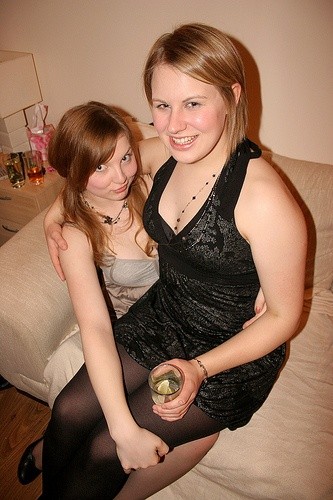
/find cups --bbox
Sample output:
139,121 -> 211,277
3,154 -> 25,189
22,150 -> 45,186
148,363 -> 185,406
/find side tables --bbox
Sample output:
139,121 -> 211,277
0,161 -> 64,247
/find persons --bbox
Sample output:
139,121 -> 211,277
42,101 -> 268,499
19,22 -> 306,500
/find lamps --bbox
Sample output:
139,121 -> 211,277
0,50 -> 43,153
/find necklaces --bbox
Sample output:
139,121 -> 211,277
173,156 -> 223,254
81,195 -> 128,225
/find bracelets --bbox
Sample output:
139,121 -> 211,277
194,359 -> 208,383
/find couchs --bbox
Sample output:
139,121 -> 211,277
0,117 -> 333,500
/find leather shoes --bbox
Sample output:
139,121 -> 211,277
17,435 -> 42,486
